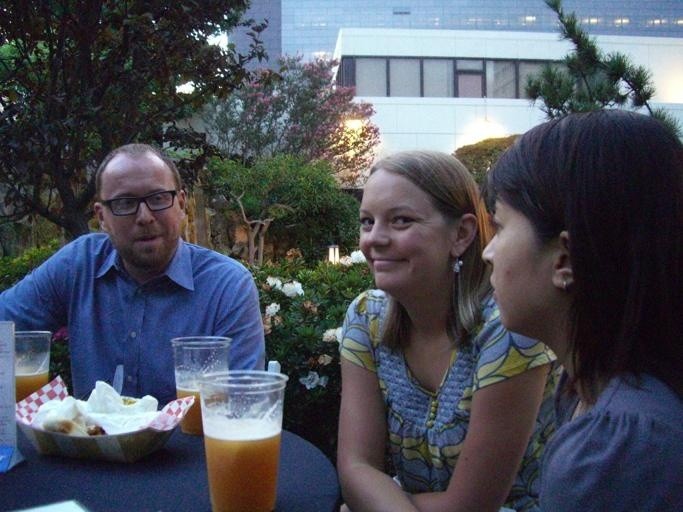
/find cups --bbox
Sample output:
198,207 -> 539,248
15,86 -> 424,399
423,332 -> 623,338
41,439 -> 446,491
11,331 -> 51,402
198,370 -> 286,511
171,334 -> 233,438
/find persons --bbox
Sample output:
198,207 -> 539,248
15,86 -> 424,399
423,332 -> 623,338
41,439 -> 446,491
480,109 -> 680,512
334,150 -> 567,512
0,142 -> 267,409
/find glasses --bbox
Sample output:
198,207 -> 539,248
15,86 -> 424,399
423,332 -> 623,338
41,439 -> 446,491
101,188 -> 180,217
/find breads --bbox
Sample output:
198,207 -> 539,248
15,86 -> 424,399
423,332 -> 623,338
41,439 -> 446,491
32,381 -> 159,439
